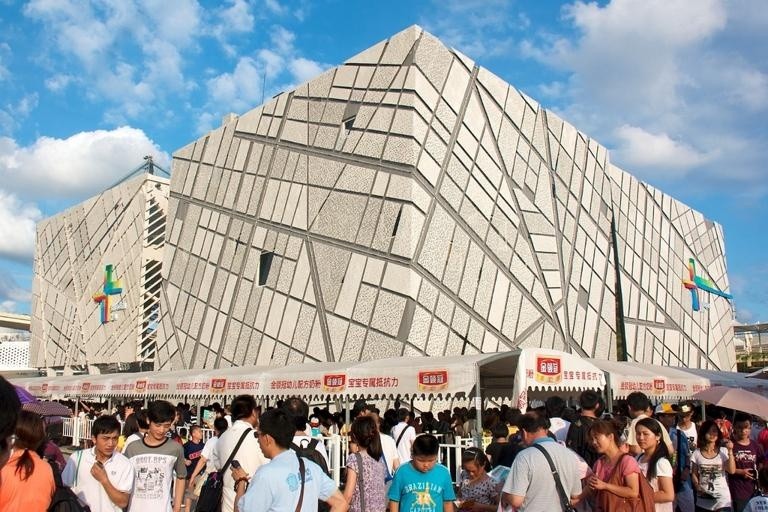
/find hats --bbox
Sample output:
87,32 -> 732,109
654,403 -> 679,414
679,401 -> 696,417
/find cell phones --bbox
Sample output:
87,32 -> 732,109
231,460 -> 240,468
97,461 -> 103,468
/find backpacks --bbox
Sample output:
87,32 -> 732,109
298,437 -> 329,479
47,461 -> 90,512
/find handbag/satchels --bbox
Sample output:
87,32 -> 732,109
674,470 -> 686,493
193,468 -> 224,512
594,451 -> 656,512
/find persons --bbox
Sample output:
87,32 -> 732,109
1,376 -> 768,511
